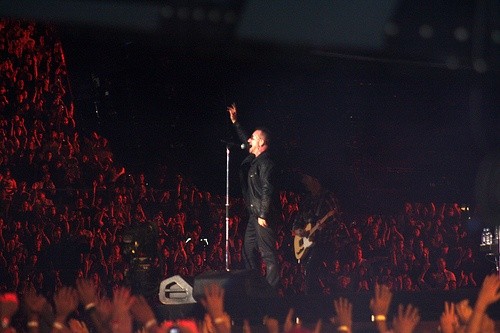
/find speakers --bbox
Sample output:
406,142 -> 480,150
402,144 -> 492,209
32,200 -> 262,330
158,269 -> 274,305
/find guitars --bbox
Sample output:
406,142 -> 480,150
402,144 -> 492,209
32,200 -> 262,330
294,208 -> 337,260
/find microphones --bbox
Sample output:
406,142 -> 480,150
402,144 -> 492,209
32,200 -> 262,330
220,139 -> 246,149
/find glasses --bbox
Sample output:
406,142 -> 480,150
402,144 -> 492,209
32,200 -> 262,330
251,135 -> 259,141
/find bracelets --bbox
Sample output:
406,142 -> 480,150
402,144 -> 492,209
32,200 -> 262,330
27,321 -> 39,326
336,326 -> 352,331
145,319 -> 154,328
213,317 -> 227,323
51,321 -> 62,330
85,303 -> 95,307
370,315 -> 386,321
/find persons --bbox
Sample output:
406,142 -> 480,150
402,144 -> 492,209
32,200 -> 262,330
226,102 -> 283,285
0,19 -> 500,333
